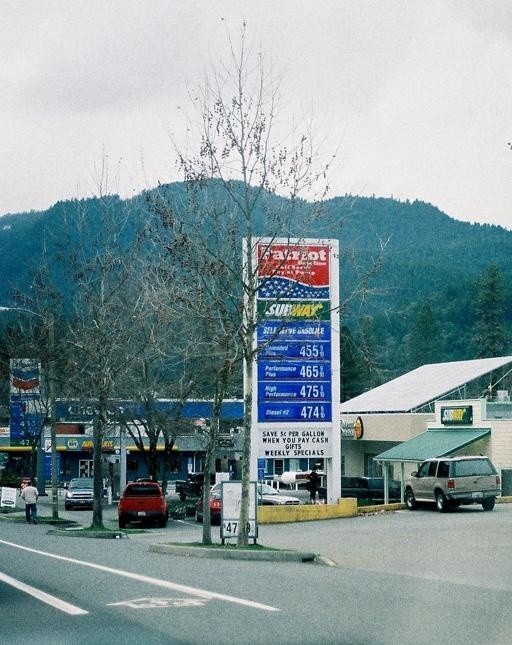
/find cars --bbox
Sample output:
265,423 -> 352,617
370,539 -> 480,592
194,482 -> 299,521
64,471 -> 95,510
118,478 -> 167,528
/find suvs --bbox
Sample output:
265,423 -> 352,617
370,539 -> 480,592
405,456 -> 500,512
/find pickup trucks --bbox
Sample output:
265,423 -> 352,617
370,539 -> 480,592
319,476 -> 401,505
176,472 -> 215,500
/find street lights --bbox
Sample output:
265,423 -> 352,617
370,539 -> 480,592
1,306 -> 57,519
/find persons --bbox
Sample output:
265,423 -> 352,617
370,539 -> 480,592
307,464 -> 319,500
21,482 -> 39,524
228,465 -> 235,480
